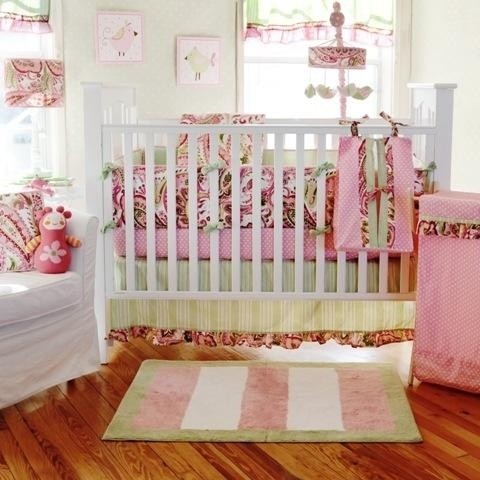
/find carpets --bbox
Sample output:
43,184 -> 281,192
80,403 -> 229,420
102,359 -> 422,443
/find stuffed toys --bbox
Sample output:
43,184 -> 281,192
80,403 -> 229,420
304,62 -> 374,104
24,205 -> 81,275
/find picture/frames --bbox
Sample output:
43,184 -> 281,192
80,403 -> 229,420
174,37 -> 224,85
94,11 -> 145,64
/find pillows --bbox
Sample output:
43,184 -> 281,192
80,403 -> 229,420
0,192 -> 44,268
177,112 -> 265,166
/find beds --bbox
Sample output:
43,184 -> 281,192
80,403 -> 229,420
100,116 -> 438,367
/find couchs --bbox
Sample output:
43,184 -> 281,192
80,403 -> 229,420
0,206 -> 101,409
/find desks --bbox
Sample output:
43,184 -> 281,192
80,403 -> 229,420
408,190 -> 480,394
